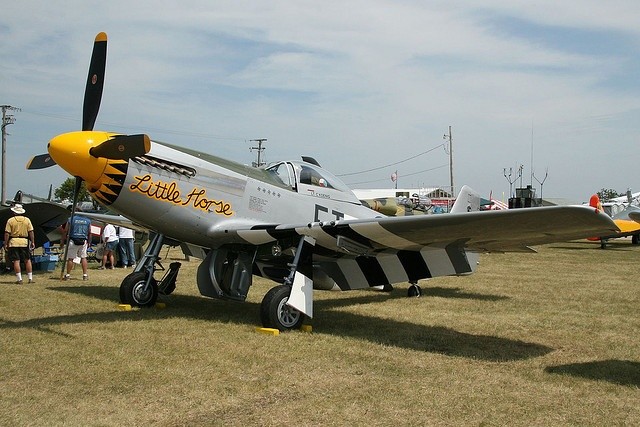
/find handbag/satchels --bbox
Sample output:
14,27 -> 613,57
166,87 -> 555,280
28,240 -> 34,253
70,237 -> 88,245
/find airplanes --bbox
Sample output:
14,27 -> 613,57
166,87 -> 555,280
595,187 -> 640,249
21,185 -> 101,212
26,31 -> 621,328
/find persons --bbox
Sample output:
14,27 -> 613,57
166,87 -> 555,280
119,215 -> 137,268
5,203 -> 36,285
59,207 -> 91,280
98,222 -> 118,270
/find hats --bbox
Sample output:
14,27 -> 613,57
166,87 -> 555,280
10,204 -> 26,214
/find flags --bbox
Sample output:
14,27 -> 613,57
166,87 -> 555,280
391,172 -> 397,182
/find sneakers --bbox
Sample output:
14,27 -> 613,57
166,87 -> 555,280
82,273 -> 89,281
28,279 -> 36,284
62,274 -> 70,281
15,280 -> 23,284
97,266 -> 106,270
